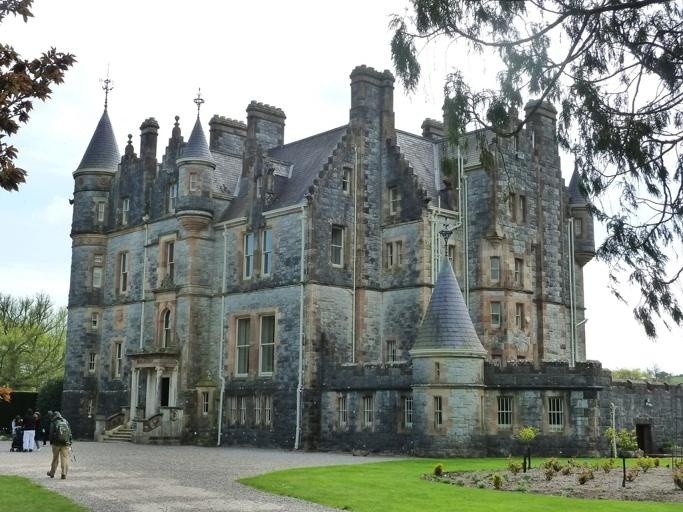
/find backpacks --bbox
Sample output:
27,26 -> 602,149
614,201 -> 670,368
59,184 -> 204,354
52,419 -> 72,446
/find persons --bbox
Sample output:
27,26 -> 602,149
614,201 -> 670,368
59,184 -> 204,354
47,411 -> 72,479
10,411 -> 53,452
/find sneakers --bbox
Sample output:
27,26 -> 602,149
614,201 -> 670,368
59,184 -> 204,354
46,471 -> 55,478
60,475 -> 66,479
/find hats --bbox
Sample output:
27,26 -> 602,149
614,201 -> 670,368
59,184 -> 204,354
50,411 -> 63,421
